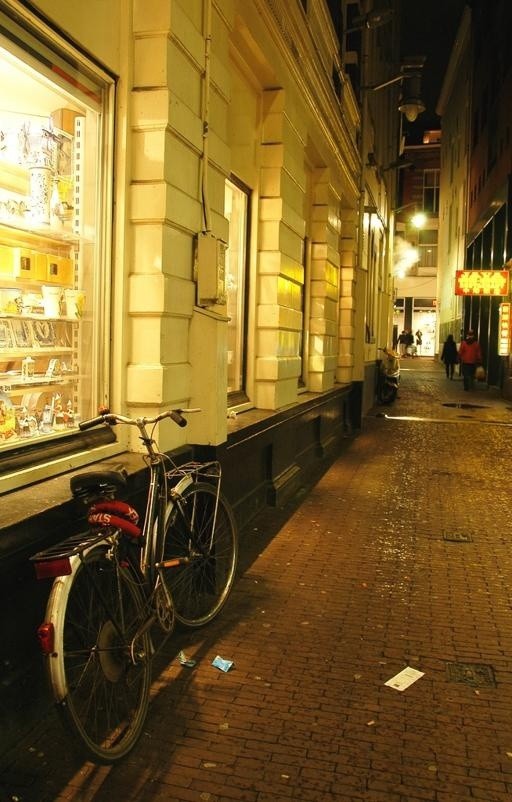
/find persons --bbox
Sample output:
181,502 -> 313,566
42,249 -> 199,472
397,329 -> 423,358
457,329 -> 483,390
441,334 -> 458,380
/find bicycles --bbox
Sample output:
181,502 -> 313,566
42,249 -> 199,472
30,409 -> 238,764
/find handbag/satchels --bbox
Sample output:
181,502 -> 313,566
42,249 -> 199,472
475,366 -> 485,380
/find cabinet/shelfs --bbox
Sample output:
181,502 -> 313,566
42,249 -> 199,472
0,211 -> 78,400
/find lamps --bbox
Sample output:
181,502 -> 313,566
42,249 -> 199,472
355,50 -> 430,122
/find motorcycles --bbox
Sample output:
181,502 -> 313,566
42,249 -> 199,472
377,347 -> 401,406
